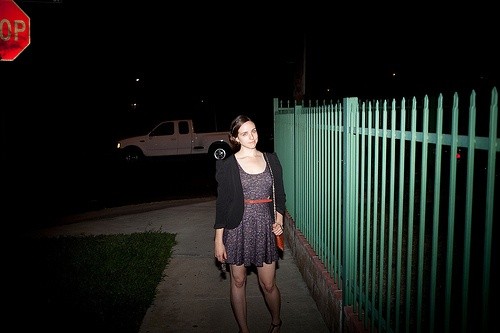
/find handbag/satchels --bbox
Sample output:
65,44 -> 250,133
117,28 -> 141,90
276,233 -> 285,251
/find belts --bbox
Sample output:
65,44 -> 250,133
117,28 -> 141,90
244,199 -> 273,204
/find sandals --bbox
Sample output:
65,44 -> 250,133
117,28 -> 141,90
268,320 -> 282,333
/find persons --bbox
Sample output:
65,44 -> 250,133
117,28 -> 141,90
213,114 -> 288,333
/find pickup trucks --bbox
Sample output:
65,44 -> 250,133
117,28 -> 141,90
112,119 -> 237,161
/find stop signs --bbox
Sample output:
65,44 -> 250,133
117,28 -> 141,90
0,0 -> 31,62
